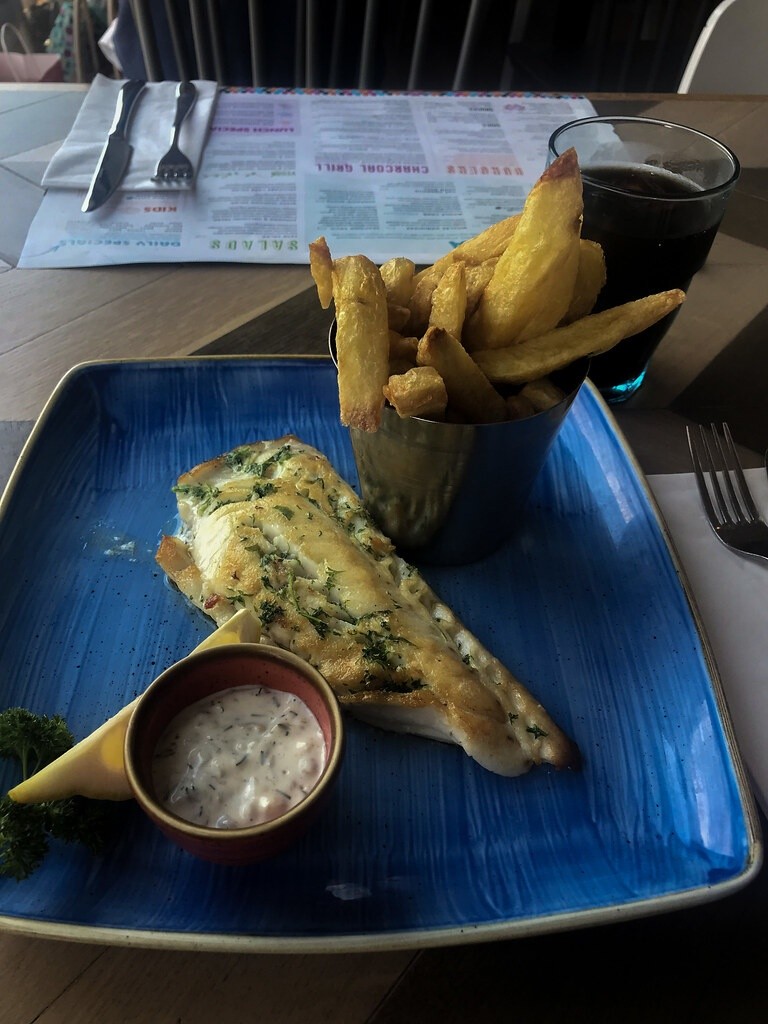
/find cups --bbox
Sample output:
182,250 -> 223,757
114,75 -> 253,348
329,307 -> 591,568
545,114 -> 743,402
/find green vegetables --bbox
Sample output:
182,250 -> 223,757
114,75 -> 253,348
0,704 -> 79,877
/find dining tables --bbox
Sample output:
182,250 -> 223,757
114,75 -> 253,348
1,83 -> 768,1023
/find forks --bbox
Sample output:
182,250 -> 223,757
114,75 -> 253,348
149,81 -> 194,183
685,422 -> 768,564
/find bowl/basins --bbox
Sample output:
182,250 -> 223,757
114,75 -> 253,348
123,642 -> 344,864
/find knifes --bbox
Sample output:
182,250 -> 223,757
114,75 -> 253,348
81,81 -> 145,214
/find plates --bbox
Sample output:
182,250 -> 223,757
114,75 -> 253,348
0,353 -> 764,956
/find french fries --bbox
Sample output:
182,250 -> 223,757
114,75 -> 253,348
304,148 -> 686,431
